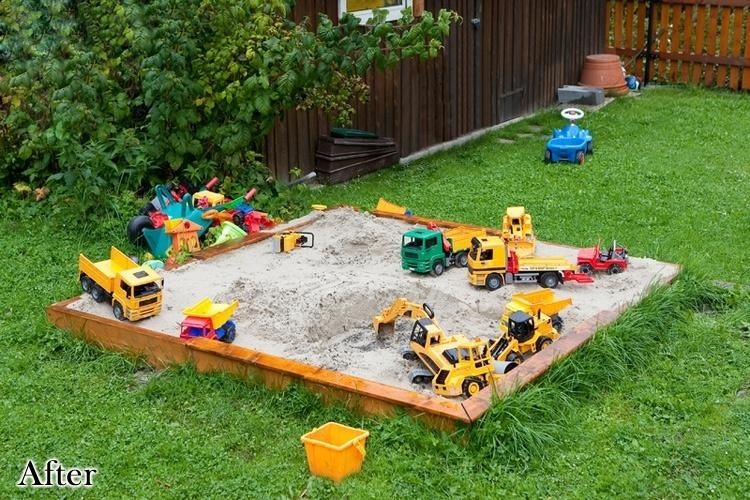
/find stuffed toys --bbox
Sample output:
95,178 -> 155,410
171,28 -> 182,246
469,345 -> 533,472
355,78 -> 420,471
372,197 -> 628,398
78,177 -> 314,344
545,108 -> 593,165
